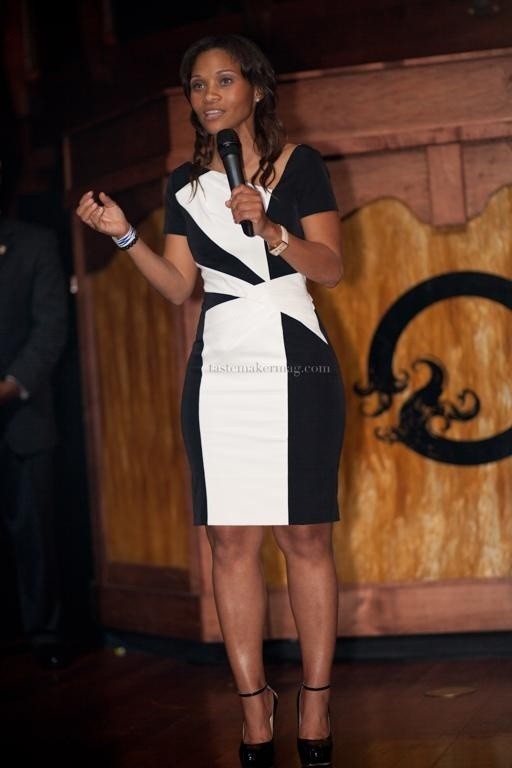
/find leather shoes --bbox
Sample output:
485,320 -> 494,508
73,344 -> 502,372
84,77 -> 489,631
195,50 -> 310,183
239,685 -> 279,768
295,685 -> 334,768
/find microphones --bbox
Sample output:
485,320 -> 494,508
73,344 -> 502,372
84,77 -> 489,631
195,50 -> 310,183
217,128 -> 254,237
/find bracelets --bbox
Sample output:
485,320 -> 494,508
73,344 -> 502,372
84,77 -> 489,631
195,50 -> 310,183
267,225 -> 288,257
111,225 -> 138,249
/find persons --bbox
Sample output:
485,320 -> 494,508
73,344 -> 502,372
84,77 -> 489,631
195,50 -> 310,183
0,218 -> 73,667
76,35 -> 348,767
